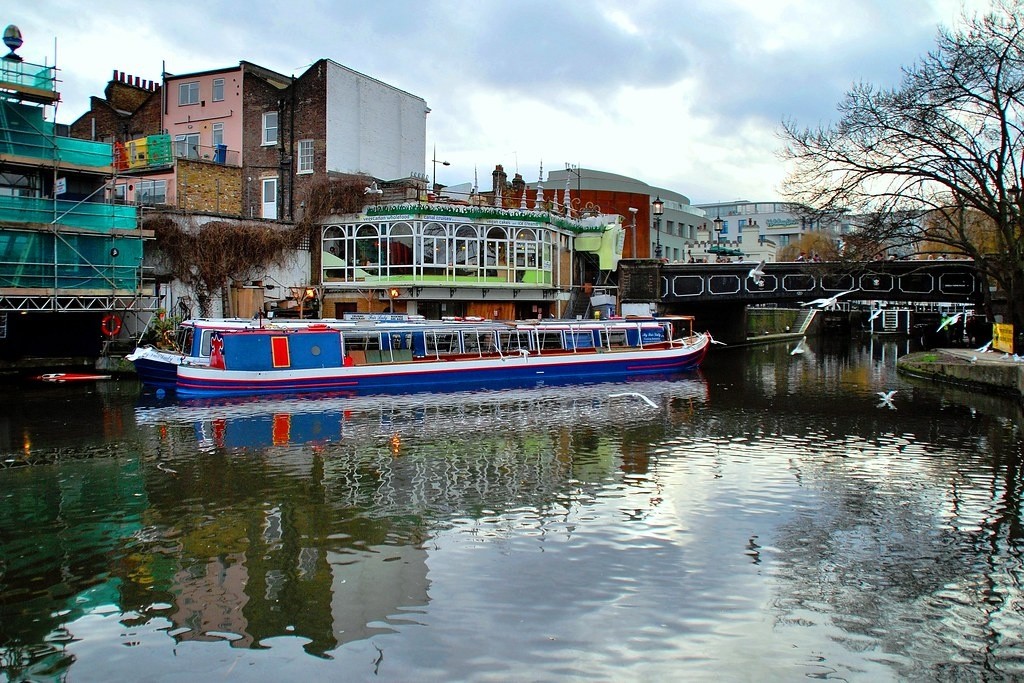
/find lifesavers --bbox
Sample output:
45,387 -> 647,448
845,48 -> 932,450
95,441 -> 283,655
102,314 -> 121,336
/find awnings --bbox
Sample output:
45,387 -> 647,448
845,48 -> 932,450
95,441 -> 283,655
704,245 -> 745,256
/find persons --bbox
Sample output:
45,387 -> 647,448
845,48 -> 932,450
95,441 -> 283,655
877,254 -> 919,260
928,254 -> 973,261
687,256 -> 743,263
795,252 -> 822,262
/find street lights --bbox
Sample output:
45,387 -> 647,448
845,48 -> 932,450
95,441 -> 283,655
714,214 -> 724,263
432,159 -> 451,188
652,195 -> 665,260
566,168 -> 581,210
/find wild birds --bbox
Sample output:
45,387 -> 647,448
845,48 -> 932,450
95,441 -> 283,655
1013,353 -> 1024,362
971,355 -> 978,362
877,391 -> 898,400
868,309 -> 882,322
801,288 -> 860,308
937,312 -> 964,333
748,260 -> 766,285
999,352 -> 1009,360
974,341 -> 993,354
608,391 -> 659,410
876,400 -> 898,410
790,336 -> 806,355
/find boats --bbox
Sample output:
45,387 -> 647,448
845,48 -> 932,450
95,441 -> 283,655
126,305 -> 711,400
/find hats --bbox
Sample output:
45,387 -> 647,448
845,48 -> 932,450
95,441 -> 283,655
942,312 -> 947,317
